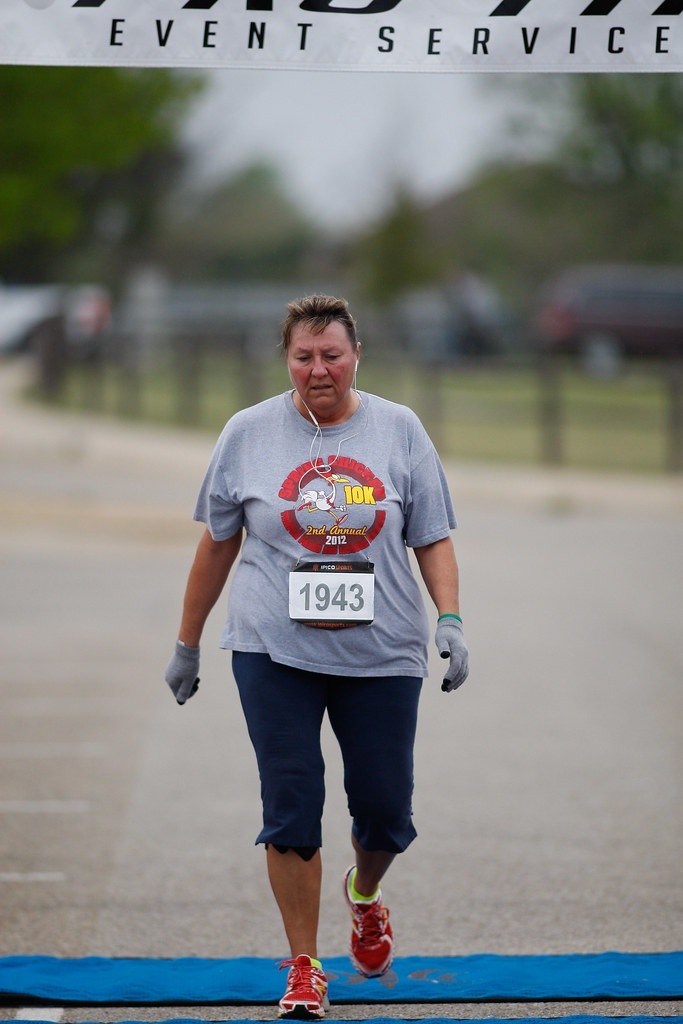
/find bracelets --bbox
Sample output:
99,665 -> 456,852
437,614 -> 462,624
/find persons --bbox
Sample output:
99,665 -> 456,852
163,295 -> 470,1020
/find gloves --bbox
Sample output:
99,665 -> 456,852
435,613 -> 470,693
164,641 -> 200,706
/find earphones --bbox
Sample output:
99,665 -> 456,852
355,360 -> 359,372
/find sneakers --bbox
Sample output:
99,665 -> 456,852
343,863 -> 395,977
278,955 -> 330,1017
